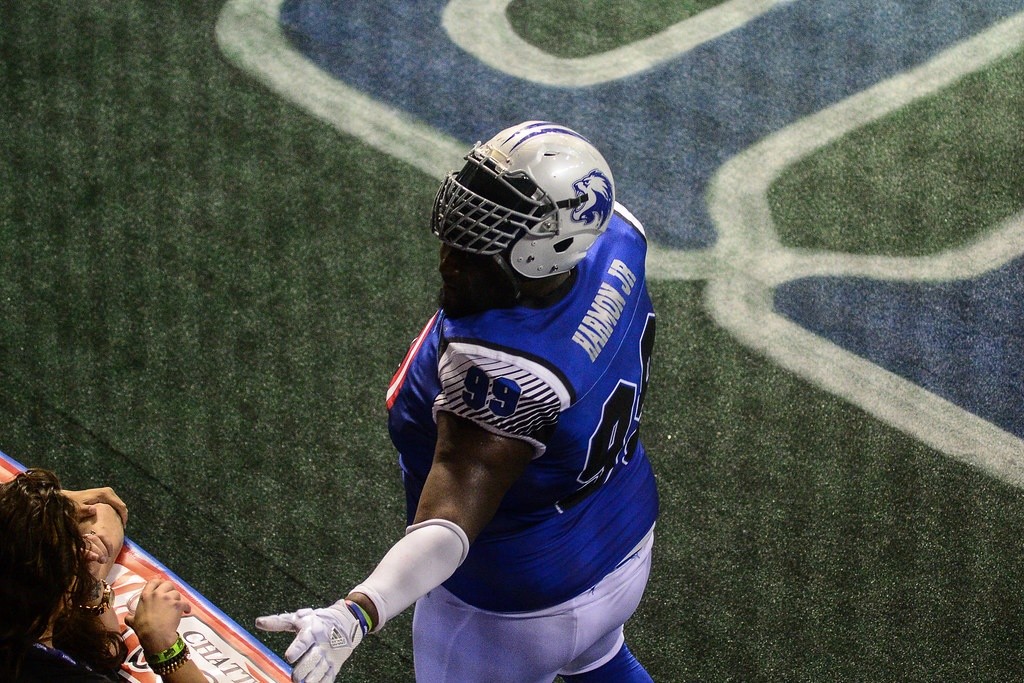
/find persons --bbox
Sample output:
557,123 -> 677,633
255,121 -> 660,683
0,469 -> 208,683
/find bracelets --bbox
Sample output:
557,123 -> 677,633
142,632 -> 185,665
345,600 -> 372,637
152,643 -> 190,676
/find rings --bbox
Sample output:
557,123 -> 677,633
84,529 -> 95,538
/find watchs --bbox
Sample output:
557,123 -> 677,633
78,579 -> 115,615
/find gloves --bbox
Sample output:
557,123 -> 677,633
254,599 -> 374,683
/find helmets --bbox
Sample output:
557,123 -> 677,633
429,121 -> 616,279
